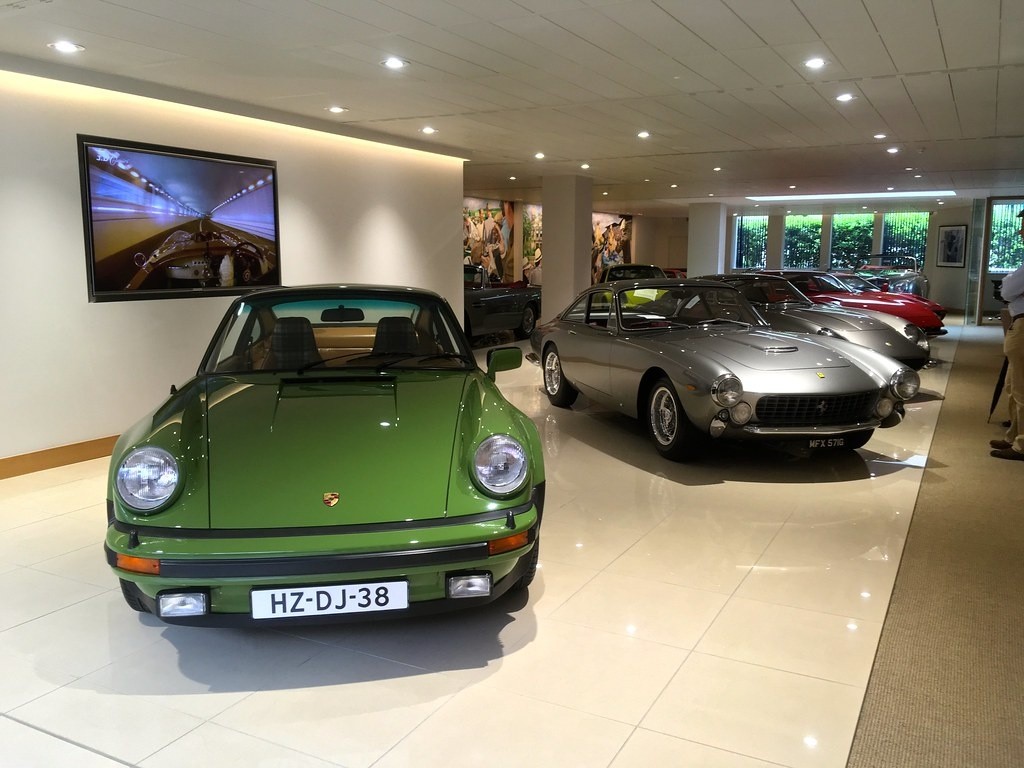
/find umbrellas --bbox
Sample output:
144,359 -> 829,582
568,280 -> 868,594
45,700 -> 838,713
987,356 -> 1009,424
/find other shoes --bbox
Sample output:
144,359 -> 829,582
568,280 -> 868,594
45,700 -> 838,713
1002,420 -> 1011,428
990,448 -> 1024,462
989,440 -> 1013,450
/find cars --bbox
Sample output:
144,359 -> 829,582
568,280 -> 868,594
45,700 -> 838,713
585,262 -> 672,310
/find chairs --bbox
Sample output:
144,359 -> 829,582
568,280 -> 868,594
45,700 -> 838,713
372,318 -> 419,357
261,317 -> 325,370
795,282 -> 808,292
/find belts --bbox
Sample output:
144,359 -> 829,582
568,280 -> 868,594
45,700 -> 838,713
1013,313 -> 1024,322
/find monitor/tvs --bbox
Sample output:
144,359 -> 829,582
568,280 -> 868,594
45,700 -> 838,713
75,132 -> 281,303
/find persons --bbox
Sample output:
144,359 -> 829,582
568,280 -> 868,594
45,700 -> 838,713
988,209 -> 1024,461
522,248 -> 543,285
590,218 -> 625,274
463,196 -> 514,284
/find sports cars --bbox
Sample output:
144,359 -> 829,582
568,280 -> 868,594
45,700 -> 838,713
766,251 -> 952,337
122,229 -> 269,291
638,272 -> 933,375
102,278 -> 550,629
463,263 -> 542,348
526,277 -> 923,461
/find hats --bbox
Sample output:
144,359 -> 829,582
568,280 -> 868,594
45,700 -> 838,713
533,248 -> 543,262
522,257 -> 532,270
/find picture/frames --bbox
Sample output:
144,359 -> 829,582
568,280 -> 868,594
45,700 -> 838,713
936,225 -> 968,268
77,134 -> 282,303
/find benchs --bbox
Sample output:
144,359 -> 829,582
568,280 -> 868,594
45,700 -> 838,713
466,280 -> 528,289
588,318 -> 707,329
